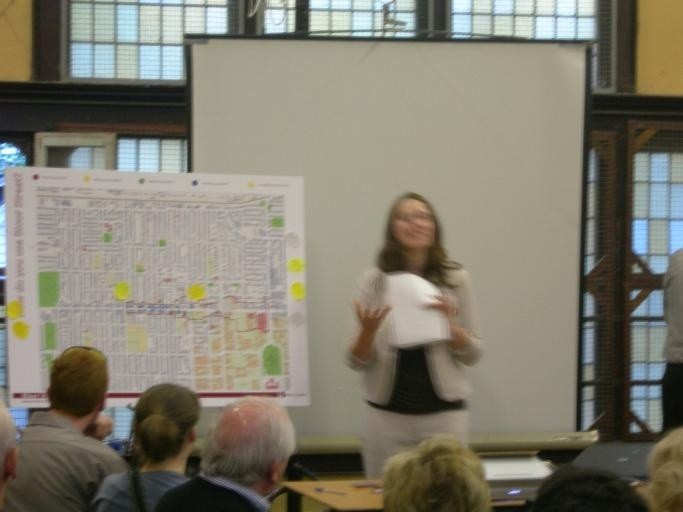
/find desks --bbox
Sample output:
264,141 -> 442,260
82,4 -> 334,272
282,479 -> 387,512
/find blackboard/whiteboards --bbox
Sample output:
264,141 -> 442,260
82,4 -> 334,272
186,36 -> 602,452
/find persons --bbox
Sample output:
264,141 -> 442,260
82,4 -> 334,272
379,433 -> 494,511
662,248 -> 683,426
345,190 -> 484,479
638,428 -> 683,510
6,344 -> 133,512
91,383 -> 201,509
0,399 -> 18,512
530,466 -> 654,510
156,393 -> 295,512
